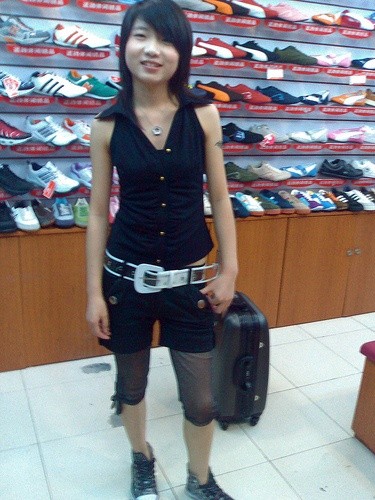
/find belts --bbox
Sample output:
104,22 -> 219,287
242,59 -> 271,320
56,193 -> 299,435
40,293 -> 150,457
103,248 -> 219,293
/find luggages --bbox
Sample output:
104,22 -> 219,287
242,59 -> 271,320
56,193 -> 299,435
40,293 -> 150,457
216,291 -> 269,430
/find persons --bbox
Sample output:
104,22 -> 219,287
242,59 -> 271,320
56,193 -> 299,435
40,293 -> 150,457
86,0 -> 238,500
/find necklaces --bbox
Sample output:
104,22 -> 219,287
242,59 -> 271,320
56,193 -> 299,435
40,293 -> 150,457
136,101 -> 168,135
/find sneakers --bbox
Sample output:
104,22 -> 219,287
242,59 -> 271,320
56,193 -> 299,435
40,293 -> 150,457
23,114 -> 78,147
0,118 -> 32,145
131,442 -> 159,500
0,67 -> 124,100
221,121 -> 375,149
186,464 -> 235,499
223,158 -> 375,218
52,23 -> 112,49
63,116 -> 92,146
0,16 -> 50,47
0,161 -> 119,231
194,79 -> 374,108
190,35 -> 375,70
203,195 -> 214,217
172,0 -> 374,34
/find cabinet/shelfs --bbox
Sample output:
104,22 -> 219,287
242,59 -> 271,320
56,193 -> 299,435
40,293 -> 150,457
0,0 -> 375,374
